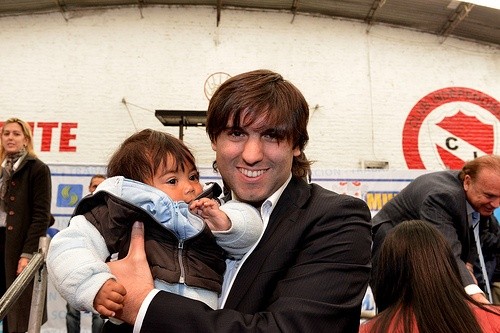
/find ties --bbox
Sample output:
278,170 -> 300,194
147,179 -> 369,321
470,211 -> 493,303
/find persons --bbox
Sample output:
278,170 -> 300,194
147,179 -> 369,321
101,69 -> 371,333
368,155 -> 500,304
46,128 -> 264,333
0,118 -> 52,333
359,220 -> 500,333
65,174 -> 106,333
